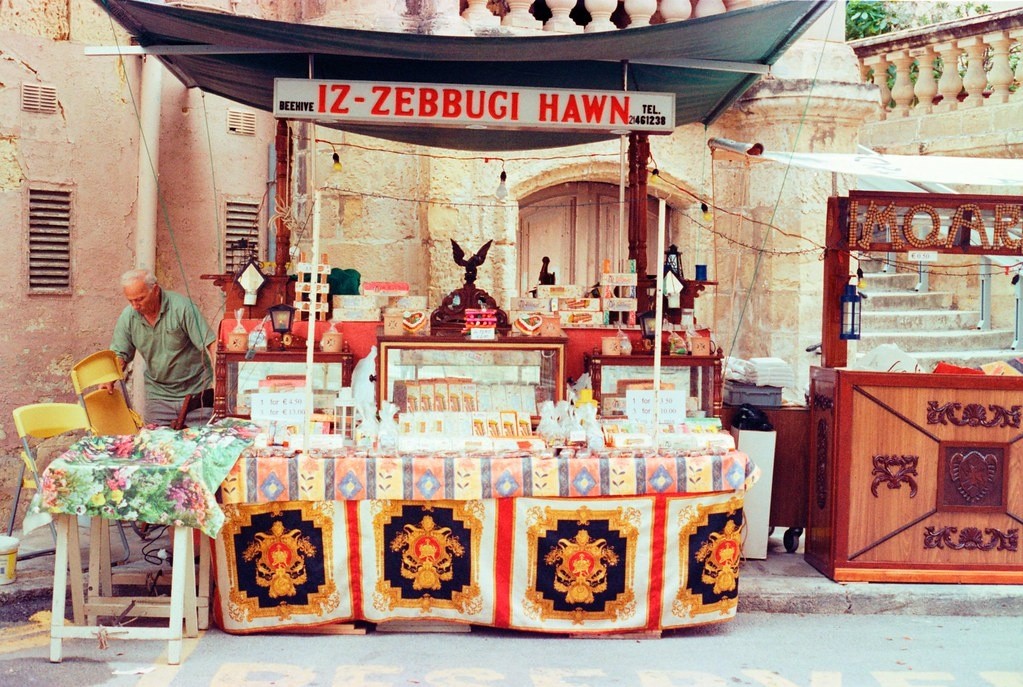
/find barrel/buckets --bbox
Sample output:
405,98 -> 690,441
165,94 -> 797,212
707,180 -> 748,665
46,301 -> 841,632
0,536 -> 20,585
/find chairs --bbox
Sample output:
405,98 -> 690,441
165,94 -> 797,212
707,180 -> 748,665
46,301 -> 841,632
71,349 -> 145,436
4,401 -> 133,571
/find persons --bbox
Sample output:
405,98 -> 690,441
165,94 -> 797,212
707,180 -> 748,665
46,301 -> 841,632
98,268 -> 218,430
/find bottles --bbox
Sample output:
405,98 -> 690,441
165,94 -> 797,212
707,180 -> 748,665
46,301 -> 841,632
333,387 -> 354,447
